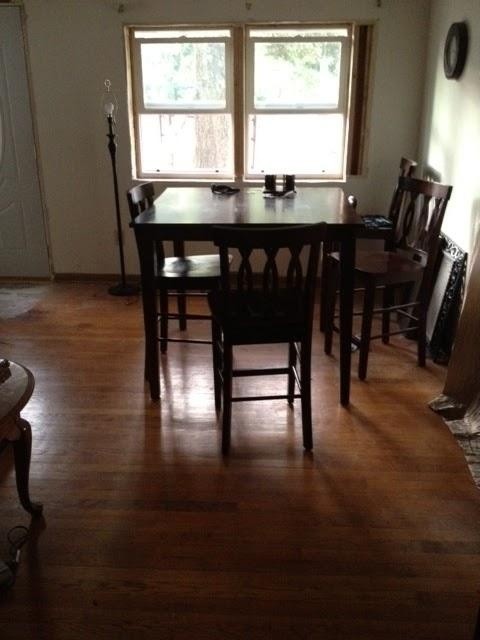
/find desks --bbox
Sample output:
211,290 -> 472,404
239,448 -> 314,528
0,360 -> 44,517
134,187 -> 360,405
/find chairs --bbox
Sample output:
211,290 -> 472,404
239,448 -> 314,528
329,156 -> 451,380
128,181 -> 232,379
202,219 -> 323,456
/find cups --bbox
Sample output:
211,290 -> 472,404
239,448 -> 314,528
265,175 -> 295,192
